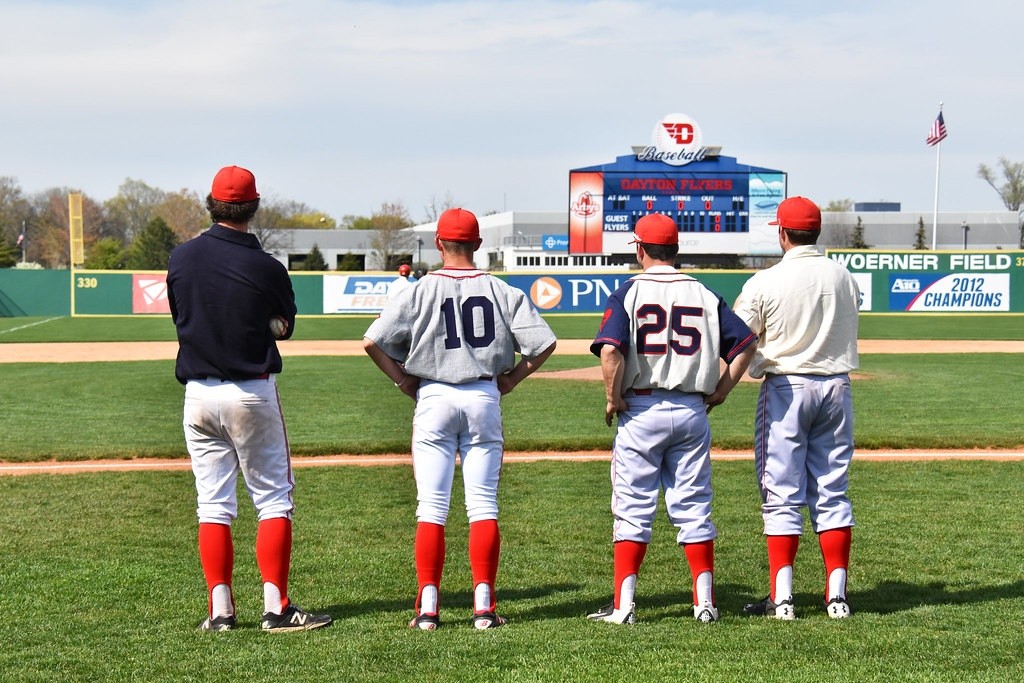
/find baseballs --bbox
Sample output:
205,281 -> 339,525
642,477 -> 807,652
269,318 -> 284,335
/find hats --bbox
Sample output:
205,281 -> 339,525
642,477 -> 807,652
437,208 -> 479,243
211,166 -> 260,203
628,213 -> 679,244
768,196 -> 822,230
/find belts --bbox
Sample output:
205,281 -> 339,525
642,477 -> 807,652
634,387 -> 651,397
192,372 -> 268,383
478,376 -> 492,382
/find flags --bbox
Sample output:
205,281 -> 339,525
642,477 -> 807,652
926,111 -> 947,148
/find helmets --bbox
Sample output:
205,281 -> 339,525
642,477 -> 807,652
414,269 -> 427,279
398,264 -> 411,277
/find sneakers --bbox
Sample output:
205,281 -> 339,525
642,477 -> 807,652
473,610 -> 509,630
260,604 -> 332,632
408,612 -> 440,630
197,613 -> 236,632
821,594 -> 851,619
688,603 -> 718,621
586,602 -> 636,626
741,594 -> 795,619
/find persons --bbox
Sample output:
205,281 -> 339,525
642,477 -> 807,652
386,264 -> 426,299
734,195 -> 860,618
361,208 -> 556,631
586,212 -> 758,625
165,165 -> 333,634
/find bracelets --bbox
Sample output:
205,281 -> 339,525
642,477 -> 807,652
395,376 -> 407,387
